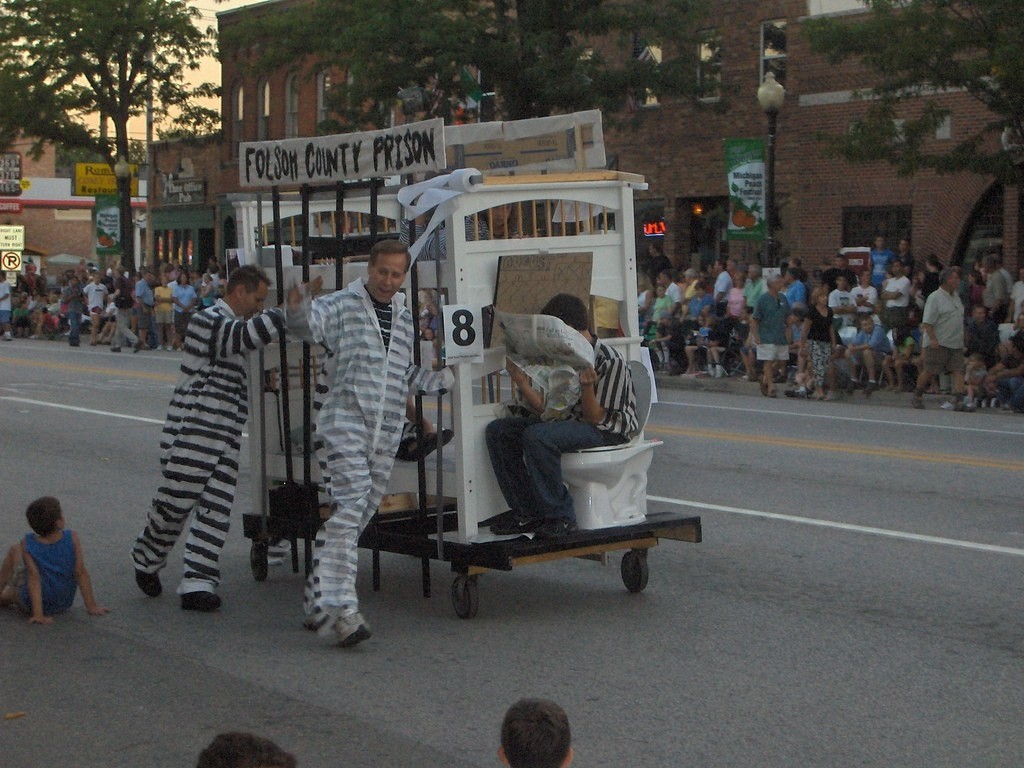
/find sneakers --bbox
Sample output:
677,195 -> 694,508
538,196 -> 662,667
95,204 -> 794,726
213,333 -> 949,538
136,567 -> 163,598
181,590 -> 223,612
302,610 -> 375,645
492,515 -> 577,539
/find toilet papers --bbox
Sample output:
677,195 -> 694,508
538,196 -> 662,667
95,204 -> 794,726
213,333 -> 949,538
447,167 -> 482,193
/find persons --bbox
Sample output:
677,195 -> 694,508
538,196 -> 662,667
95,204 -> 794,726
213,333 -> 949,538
126,263 -> 325,612
196,730 -> 297,768
0,495 -> 110,624
636,236 -> 1024,412
497,697 -> 572,768
750,273 -> 791,398
799,286 -> 836,401
0,255 -> 237,349
82,271 -> 110,345
414,287 -> 455,374
396,394 -> 454,461
154,276 -> 174,351
399,202 -> 530,262
482,293 -> 641,537
62,276 -> 85,347
135,270 -> 154,349
108,263 -> 143,353
0,271 -> 12,340
172,271 -> 198,351
911,266 -> 965,408
286,239 -> 457,645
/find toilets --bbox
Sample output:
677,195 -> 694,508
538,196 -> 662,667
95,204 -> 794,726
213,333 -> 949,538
521,358 -> 666,532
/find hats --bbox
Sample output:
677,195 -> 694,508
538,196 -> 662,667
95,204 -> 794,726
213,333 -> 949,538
682,268 -> 698,277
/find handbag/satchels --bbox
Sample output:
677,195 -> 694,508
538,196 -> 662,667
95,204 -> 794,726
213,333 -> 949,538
114,295 -> 134,308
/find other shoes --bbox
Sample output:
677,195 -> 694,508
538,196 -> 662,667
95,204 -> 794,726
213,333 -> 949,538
658,359 -> 730,379
886,380 -> 1021,414
110,347 -> 121,352
2,327 -> 112,346
761,377 -> 878,403
145,339 -> 184,352
133,341 -> 142,353
